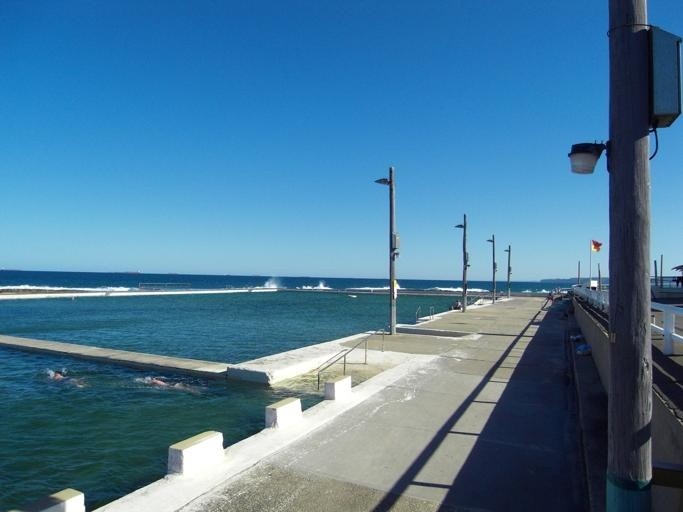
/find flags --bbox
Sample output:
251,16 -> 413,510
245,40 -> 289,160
591,240 -> 603,252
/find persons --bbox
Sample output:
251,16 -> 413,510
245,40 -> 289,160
152,376 -> 197,396
51,369 -> 85,390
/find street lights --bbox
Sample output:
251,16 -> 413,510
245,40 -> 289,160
486,233 -> 498,306
502,244 -> 514,300
453,211 -> 469,315
373,164 -> 402,336
